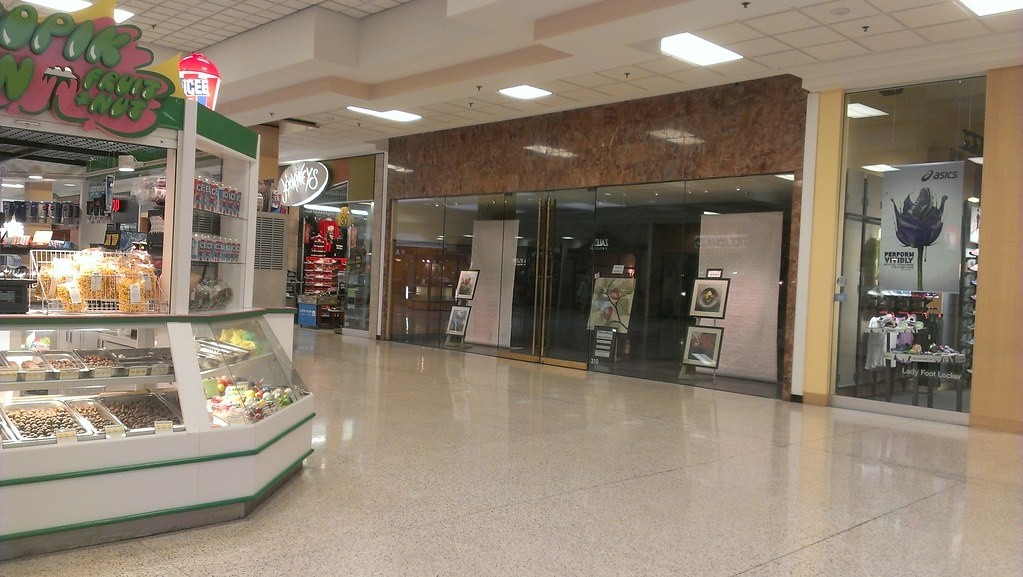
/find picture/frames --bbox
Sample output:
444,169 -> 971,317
688,277 -> 731,319
681,324 -> 724,369
454,269 -> 480,299
445,305 -> 472,337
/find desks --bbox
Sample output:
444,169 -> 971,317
882,350 -> 965,408
865,325 -> 928,402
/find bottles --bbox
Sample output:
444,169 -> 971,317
194,171 -> 243,217
191,232 -> 241,262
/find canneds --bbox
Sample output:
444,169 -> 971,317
2,201 -> 77,224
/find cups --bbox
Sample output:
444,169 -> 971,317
178,51 -> 222,111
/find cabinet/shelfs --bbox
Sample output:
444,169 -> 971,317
880,290 -> 943,322
954,201 -> 978,383
